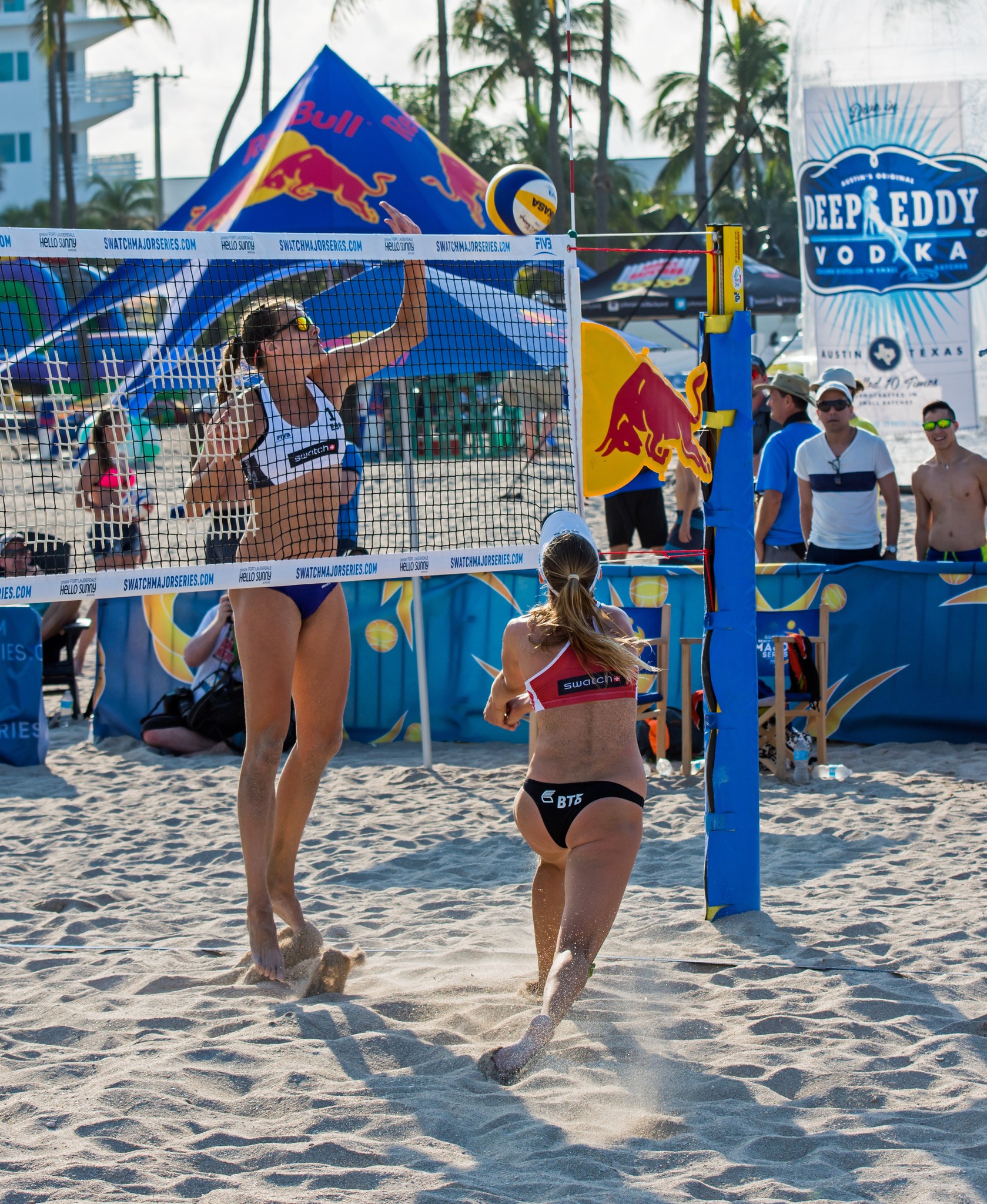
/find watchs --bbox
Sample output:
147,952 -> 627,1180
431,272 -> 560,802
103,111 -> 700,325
885,546 -> 898,553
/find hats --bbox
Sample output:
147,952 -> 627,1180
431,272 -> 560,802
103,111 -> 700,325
0,533 -> 25,550
752,354 -> 766,373
191,392 -> 218,414
537,509 -> 601,597
531,290 -> 554,305
809,367 -> 856,391
754,373 -> 816,407
816,380 -> 852,405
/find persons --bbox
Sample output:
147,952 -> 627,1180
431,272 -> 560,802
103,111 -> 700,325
0,393 -> 364,673
141,595 -> 295,758
477,531 -> 647,1085
184,199 -> 427,987
509,291 -> 562,461
604,355 -> 903,563
910,401 -> 987,562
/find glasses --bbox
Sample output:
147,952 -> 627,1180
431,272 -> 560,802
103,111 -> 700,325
922,419 -> 955,431
761,388 -> 771,398
816,400 -> 850,412
1,548 -> 25,557
254,315 -> 314,367
828,458 -> 841,485
752,370 -> 761,380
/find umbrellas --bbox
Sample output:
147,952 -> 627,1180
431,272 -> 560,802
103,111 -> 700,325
126,262 -> 666,553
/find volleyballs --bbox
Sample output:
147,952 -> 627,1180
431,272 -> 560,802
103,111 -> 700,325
484,163 -> 558,237
122,486 -> 154,519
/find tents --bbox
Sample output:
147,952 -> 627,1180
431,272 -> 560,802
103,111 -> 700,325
549,214 -> 802,321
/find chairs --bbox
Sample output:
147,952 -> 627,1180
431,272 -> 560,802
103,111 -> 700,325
0,531 -> 94,721
617,602 -> 671,776
678,601 -> 831,778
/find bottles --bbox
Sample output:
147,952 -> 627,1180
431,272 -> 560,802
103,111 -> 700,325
793,737 -> 809,784
812,764 -> 853,779
680,759 -> 705,775
59,690 -> 73,728
643,763 -> 651,778
656,759 -> 673,776
